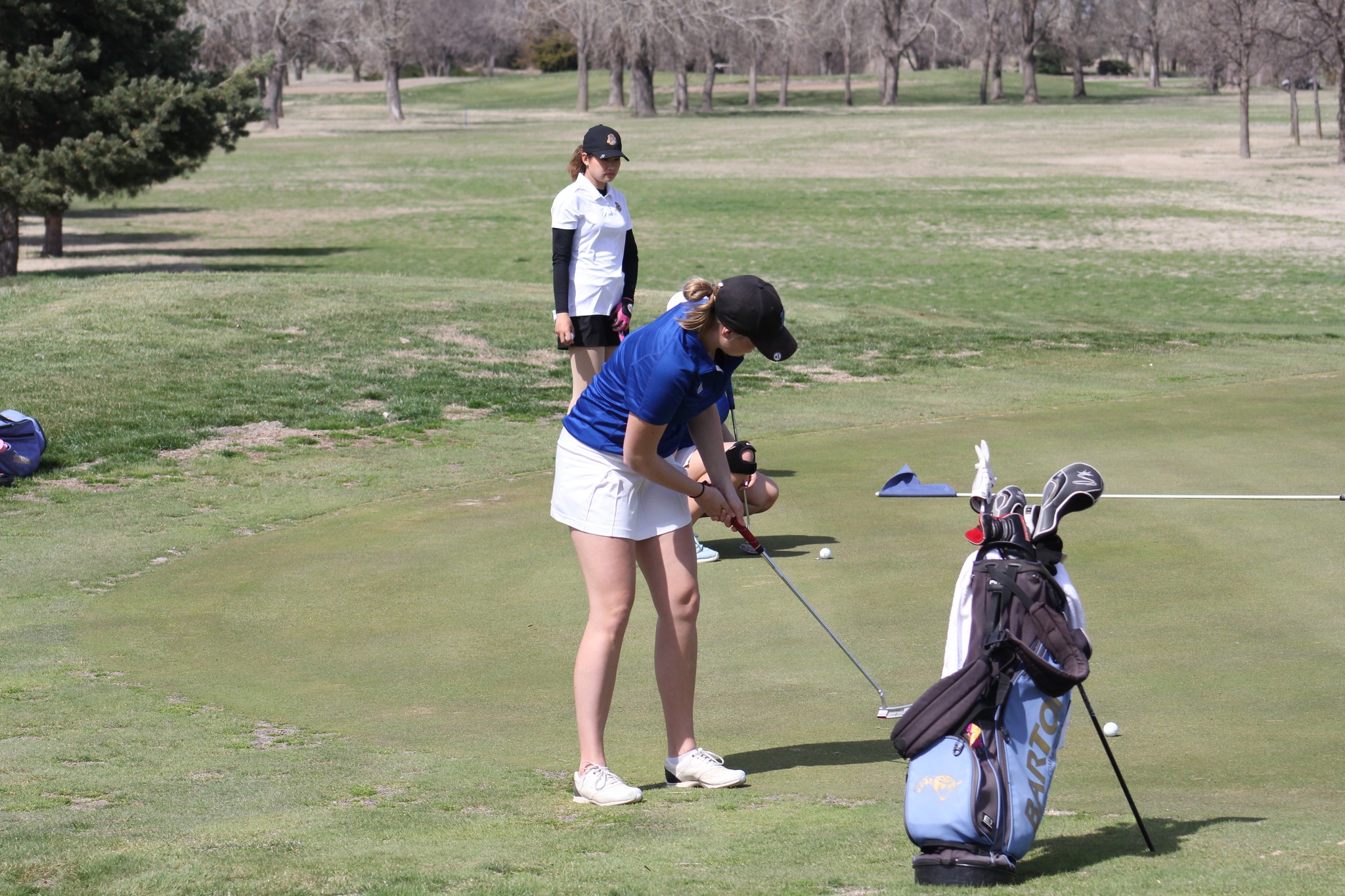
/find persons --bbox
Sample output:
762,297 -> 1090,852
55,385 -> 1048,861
665,290 -> 780,563
551,124 -> 639,415
548,275 -> 798,807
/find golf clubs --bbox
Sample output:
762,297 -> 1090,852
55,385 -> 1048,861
728,515 -> 914,718
1017,503 -> 1156,852
726,376 -> 766,555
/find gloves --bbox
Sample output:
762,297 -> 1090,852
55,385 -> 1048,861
969,439 -> 997,514
609,298 -> 634,332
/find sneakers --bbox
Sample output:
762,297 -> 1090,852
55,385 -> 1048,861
664,747 -> 746,788
694,537 -> 719,563
572,761 -> 642,806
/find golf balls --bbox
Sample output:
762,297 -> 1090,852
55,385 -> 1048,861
819,547 -> 831,559
1103,722 -> 1118,737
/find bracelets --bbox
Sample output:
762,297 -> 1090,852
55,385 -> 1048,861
689,482 -> 705,499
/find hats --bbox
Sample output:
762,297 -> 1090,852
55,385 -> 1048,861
667,291 -> 709,312
714,275 -> 797,362
582,124 -> 629,162
